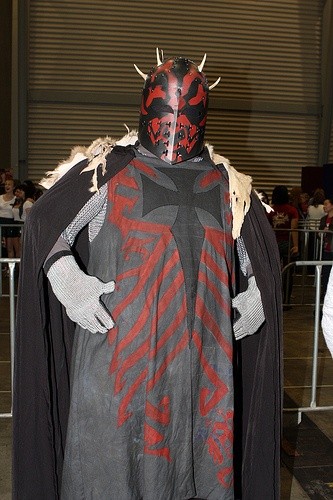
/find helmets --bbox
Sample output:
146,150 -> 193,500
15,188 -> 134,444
134,47 -> 221,166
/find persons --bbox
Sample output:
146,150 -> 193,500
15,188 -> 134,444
0,168 -> 333,358
11,45 -> 284,500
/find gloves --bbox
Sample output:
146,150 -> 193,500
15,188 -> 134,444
231,274 -> 265,342
47,255 -> 115,334
